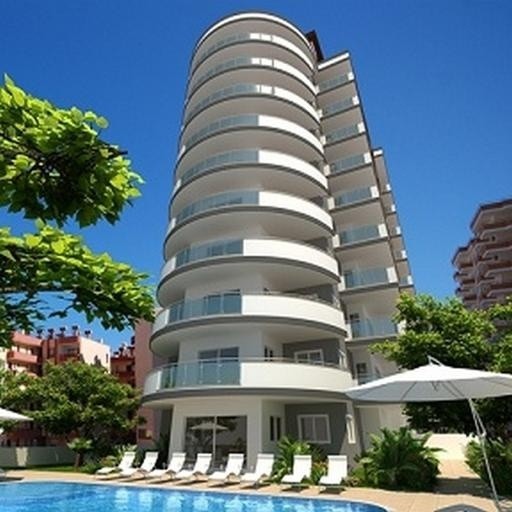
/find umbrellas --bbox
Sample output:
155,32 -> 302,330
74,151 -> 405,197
188,421 -> 229,453
345,355 -> 512,505
0,407 -> 34,423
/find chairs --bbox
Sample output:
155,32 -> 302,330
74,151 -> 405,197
96,451 -> 349,487
